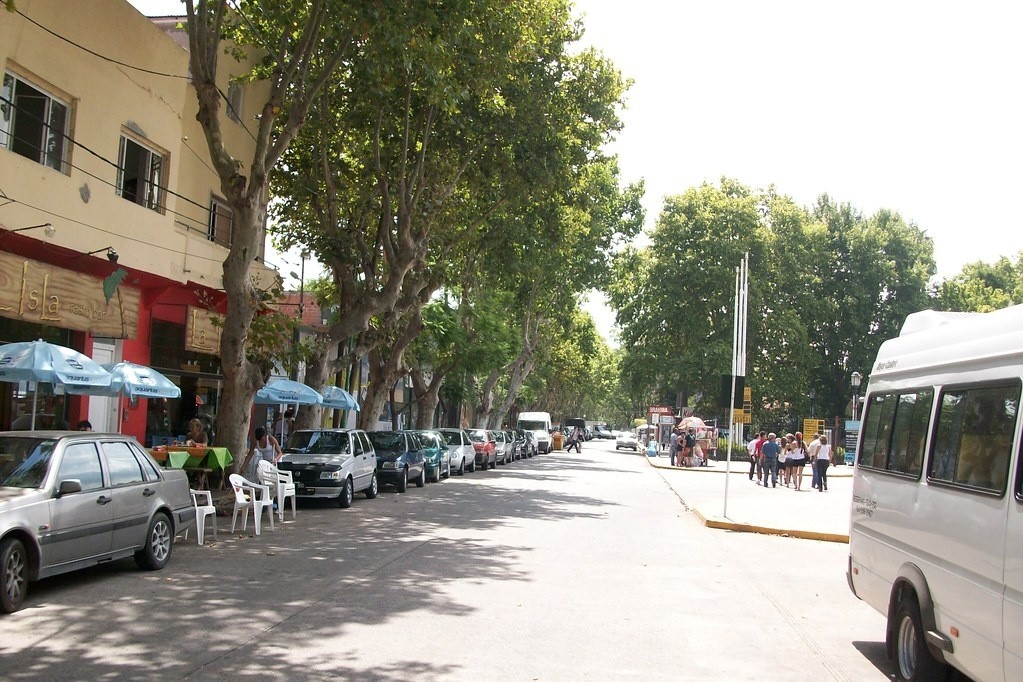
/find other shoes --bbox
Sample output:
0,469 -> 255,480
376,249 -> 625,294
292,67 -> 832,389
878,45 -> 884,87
798,485 -> 801,490
818,488 -> 822,492
274,509 -> 287,514
577,451 -> 581,453
811,484 -> 815,488
567,450 -> 571,453
824,486 -> 828,490
757,481 -> 760,485
773,483 -> 776,487
794,484 -> 797,489
780,481 -> 783,485
749,477 -> 752,480
764,483 -> 768,487
786,483 -> 789,487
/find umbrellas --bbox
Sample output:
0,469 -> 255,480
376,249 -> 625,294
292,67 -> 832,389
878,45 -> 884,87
637,423 -> 658,429
254,378 -> 324,447
0,338 -> 112,430
307,385 -> 360,412
53,359 -> 182,434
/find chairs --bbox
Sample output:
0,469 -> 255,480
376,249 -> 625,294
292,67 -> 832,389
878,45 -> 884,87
229,473 -> 274,535
255,459 -> 295,522
173,490 -> 216,546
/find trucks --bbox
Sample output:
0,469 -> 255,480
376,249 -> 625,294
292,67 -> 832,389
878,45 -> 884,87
585,420 -> 611,439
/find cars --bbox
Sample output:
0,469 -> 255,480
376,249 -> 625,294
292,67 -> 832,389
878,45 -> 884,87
432,427 -> 476,476
463,428 -> 498,471
562,418 -> 592,447
506,428 -> 538,462
616,431 -> 637,451
609,430 -> 621,439
366,430 -> 426,494
0,430 -> 196,616
493,431 -> 513,466
412,430 -> 450,483
276,428 -> 379,509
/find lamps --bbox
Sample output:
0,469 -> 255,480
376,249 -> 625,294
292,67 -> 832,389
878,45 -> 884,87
84,246 -> 118,265
8,223 -> 55,237
266,302 -> 304,312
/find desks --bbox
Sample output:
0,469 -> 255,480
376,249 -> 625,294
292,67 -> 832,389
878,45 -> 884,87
146,449 -> 190,468
163,446 -> 232,469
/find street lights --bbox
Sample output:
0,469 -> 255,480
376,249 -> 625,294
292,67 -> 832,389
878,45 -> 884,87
289,270 -> 303,383
851,370 -> 862,421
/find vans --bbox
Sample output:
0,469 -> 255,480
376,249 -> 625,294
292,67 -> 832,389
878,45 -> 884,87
515,412 -> 555,454
847,303 -> 1023,682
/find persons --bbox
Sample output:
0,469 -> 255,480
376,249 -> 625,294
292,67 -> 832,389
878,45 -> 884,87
57,420 -> 72,430
184,419 -> 209,447
271,408 -> 296,445
807,433 -> 820,489
669,427 -> 678,466
812,435 -> 836,492
777,433 -> 795,486
566,426 -> 585,453
77,420 -> 92,430
790,431 -> 811,491
958,396 -> 1007,489
680,428 -> 695,465
696,427 -> 706,439
237,426 -> 283,514
677,431 -> 685,466
747,431 -> 781,488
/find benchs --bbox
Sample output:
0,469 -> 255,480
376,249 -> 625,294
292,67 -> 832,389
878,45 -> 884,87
180,463 -> 236,491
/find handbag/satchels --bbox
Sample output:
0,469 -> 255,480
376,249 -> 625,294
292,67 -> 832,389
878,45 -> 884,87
804,451 -> 808,461
